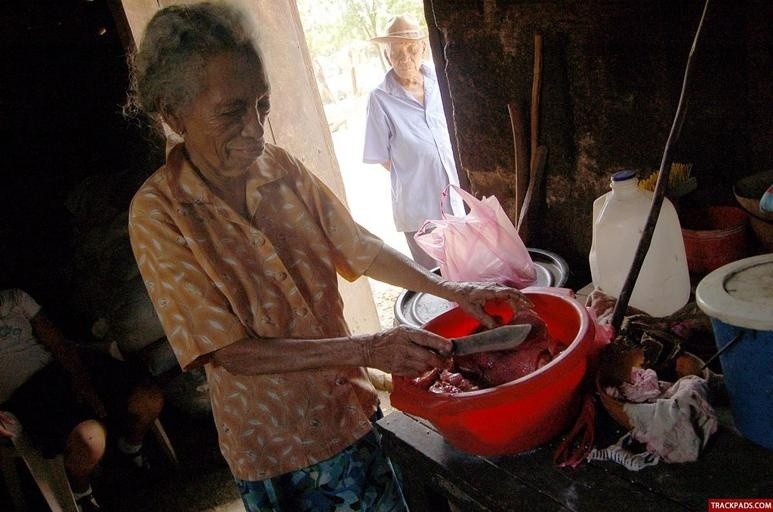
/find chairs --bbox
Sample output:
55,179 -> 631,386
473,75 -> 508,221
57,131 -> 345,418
0,340 -> 180,512
91,278 -> 216,464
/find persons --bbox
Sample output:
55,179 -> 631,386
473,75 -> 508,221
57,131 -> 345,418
0,285 -> 166,509
121,0 -> 534,512
360,16 -> 465,272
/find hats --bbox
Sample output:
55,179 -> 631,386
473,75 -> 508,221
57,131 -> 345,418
368,13 -> 430,42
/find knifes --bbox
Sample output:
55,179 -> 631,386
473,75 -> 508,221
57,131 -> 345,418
444,324 -> 532,358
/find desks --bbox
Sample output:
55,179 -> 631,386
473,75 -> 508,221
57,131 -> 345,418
374,281 -> 771,512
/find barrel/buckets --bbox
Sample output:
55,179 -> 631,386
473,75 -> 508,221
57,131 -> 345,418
694,251 -> 773,450
679,204 -> 750,272
